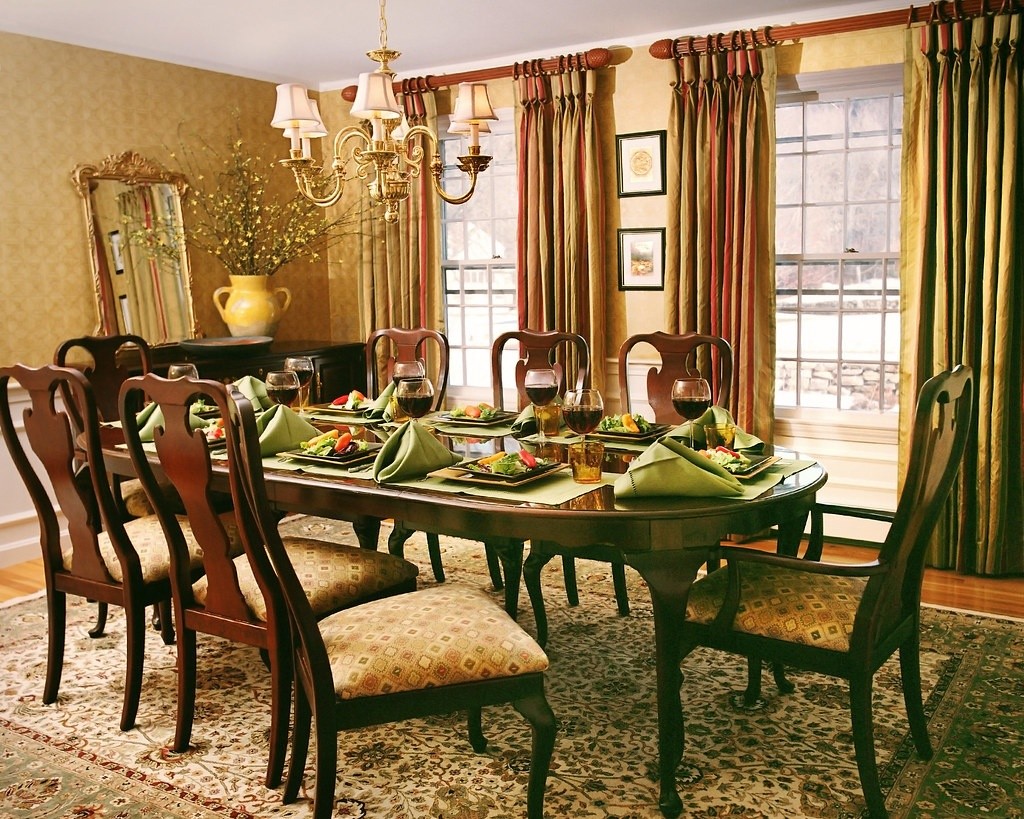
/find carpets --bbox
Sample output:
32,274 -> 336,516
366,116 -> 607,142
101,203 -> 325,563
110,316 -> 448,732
1,511 -> 1024,819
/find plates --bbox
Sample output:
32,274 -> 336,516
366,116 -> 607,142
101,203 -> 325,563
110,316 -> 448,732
314,403 -> 368,410
206,438 -> 226,443
449,454 -> 561,479
597,423 -> 671,436
193,406 -> 220,414
179,336 -> 274,353
441,411 -> 520,422
723,455 -> 773,475
287,443 -> 383,460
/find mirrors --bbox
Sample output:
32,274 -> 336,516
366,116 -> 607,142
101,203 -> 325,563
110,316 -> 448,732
70,149 -> 206,354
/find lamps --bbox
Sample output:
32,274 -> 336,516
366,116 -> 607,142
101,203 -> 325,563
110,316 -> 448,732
272,0 -> 500,224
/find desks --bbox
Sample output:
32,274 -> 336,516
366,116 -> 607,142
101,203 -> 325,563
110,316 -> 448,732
73,403 -> 830,819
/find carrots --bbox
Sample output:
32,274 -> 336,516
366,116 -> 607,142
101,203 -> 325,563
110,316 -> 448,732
334,433 -> 351,449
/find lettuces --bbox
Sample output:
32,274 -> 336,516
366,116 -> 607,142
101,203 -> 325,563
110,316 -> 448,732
466,452 -> 548,474
698,448 -> 753,473
450,404 -> 500,418
599,414 -> 652,432
352,395 -> 362,406
299,437 -> 368,457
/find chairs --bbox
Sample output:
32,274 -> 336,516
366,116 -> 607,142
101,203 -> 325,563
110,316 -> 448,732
1,326 -> 973,819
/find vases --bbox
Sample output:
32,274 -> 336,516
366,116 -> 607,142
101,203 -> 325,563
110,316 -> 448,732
214,273 -> 291,338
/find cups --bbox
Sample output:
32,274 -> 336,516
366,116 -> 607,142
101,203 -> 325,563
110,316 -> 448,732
569,442 -> 604,483
291,386 -> 309,414
704,424 -> 736,451
265,372 -> 300,405
389,396 -> 409,422
533,404 -> 561,436
168,364 -> 199,381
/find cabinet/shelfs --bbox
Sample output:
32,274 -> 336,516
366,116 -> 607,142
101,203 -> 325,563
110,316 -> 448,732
66,339 -> 370,474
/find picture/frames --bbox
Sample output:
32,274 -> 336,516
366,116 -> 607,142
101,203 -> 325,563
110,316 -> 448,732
106,230 -> 135,335
617,227 -> 665,292
615,130 -> 667,198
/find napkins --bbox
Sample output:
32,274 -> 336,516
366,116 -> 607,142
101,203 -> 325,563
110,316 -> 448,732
372,418 -> 463,484
614,435 -> 745,498
659,405 -> 767,454
256,405 -> 324,459
228,375 -> 275,416
135,399 -> 208,443
363,380 -> 399,422
511,394 -> 566,432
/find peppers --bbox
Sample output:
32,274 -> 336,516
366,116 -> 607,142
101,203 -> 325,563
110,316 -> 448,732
715,446 -> 740,459
622,414 -> 640,433
206,418 -> 224,440
479,403 -> 494,409
699,449 -> 711,458
308,429 -> 339,443
477,452 -> 508,464
466,405 -> 480,417
332,395 -> 349,404
519,450 -> 536,467
352,390 -> 365,400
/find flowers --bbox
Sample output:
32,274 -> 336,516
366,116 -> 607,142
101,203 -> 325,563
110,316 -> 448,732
91,114 -> 386,277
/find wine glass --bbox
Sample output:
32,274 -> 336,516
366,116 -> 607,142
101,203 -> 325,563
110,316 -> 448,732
398,378 -> 434,423
392,361 -> 425,421
562,390 -> 604,476
284,357 -> 314,418
672,378 -> 711,451
525,369 -> 558,442
